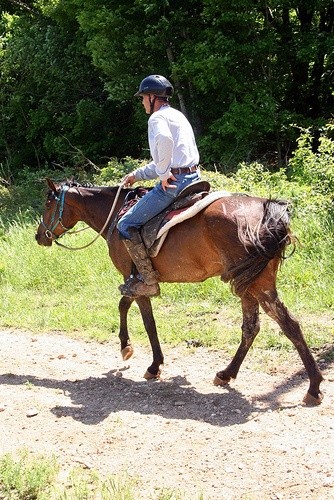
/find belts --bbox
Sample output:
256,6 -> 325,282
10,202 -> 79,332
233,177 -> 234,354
170,165 -> 198,174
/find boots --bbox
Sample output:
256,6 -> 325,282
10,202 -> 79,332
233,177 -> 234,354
117,233 -> 160,297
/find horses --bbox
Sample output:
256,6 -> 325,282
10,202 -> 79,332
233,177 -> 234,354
34,178 -> 326,405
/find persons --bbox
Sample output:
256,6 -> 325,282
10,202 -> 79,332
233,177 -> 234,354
115,73 -> 202,298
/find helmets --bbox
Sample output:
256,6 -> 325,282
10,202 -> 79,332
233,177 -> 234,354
134,74 -> 175,98
